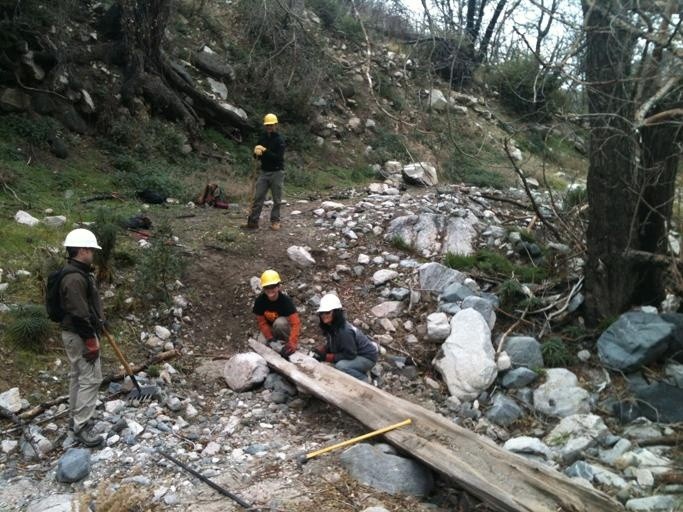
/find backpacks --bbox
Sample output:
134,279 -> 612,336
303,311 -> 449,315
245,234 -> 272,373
45,270 -> 89,323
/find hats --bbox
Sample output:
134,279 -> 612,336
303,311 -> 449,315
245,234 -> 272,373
315,293 -> 342,313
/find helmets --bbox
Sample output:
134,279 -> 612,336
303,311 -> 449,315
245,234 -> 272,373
62,228 -> 103,250
260,269 -> 282,289
263,113 -> 279,126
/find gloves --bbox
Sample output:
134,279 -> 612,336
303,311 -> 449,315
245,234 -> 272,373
254,144 -> 267,156
80,332 -> 100,364
280,343 -> 296,356
313,345 -> 334,362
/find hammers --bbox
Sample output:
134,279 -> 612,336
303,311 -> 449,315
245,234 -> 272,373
300,418 -> 413,463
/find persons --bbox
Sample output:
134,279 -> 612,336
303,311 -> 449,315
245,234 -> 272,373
250,269 -> 302,358
45,227 -> 108,447
238,112 -> 286,231
312,293 -> 378,386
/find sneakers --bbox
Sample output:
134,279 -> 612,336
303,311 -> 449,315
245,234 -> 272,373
271,222 -> 281,230
73,429 -> 104,447
240,223 -> 258,230
